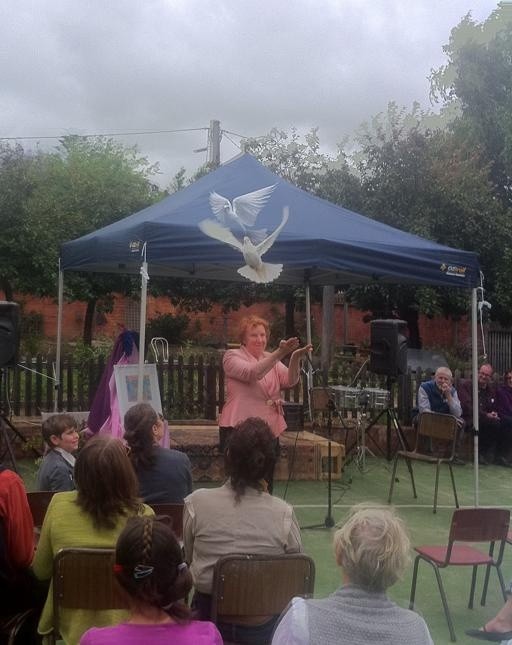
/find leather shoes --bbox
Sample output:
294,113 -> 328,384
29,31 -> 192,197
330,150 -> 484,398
473,454 -> 488,464
493,456 -> 510,467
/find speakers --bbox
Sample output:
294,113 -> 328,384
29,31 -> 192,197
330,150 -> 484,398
0,300 -> 21,367
370,320 -> 408,376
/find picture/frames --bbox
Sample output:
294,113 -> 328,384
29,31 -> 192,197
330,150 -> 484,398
113,363 -> 163,424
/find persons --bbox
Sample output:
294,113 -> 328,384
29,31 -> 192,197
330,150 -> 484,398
270,502 -> 433,645
182,416 -> 303,629
122,402 -> 192,505
465,580 -> 512,644
0,462 -> 34,587
497,370 -> 512,419
219,315 -> 314,495
34,435 -> 155,645
412,366 -> 465,466
462,363 -> 512,467
38,414 -> 79,492
79,515 -> 223,644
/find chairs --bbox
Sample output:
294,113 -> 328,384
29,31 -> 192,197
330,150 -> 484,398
150,336 -> 179,365
409,508 -> 510,642
480,522 -> 512,607
210,552 -> 316,625
47,547 -> 116,644
389,412 -> 460,515
148,503 -> 184,543
26,490 -> 57,527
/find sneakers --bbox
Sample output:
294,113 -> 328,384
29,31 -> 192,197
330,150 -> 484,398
454,455 -> 465,465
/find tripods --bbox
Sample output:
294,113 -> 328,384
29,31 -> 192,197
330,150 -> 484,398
342,378 -> 418,498
296,337 -> 347,532
0,411 -> 42,481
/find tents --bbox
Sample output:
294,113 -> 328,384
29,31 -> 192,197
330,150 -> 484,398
51,152 -> 483,508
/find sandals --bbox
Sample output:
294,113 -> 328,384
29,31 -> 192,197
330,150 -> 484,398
465,626 -> 511,641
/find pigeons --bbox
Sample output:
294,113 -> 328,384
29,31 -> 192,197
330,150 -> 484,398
198,206 -> 290,284
208,184 -> 276,231
